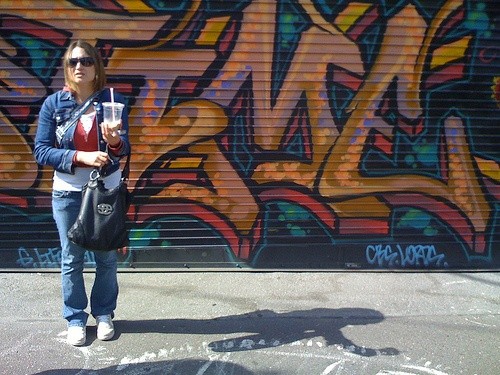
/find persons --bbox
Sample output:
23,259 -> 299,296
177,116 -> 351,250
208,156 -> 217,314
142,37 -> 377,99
35,41 -> 131,345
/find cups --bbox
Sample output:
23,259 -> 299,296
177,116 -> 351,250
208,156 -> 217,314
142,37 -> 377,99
102,102 -> 124,134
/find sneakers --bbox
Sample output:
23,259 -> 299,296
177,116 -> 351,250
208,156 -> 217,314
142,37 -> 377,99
95,314 -> 115,340
67,325 -> 86,346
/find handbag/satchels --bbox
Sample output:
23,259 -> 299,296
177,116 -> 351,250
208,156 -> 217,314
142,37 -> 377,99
67,139 -> 131,250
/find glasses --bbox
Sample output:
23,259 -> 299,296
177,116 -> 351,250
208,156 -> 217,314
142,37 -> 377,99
66,57 -> 94,67
